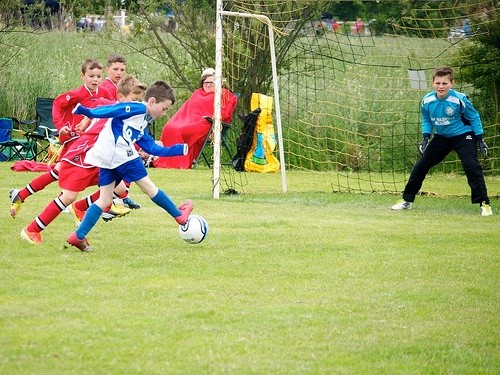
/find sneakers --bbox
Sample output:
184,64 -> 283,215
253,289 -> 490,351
113,197 -> 141,209
20,225 -> 41,244
9,189 -> 25,219
101,204 -> 131,222
175,199 -> 193,226
480,201 -> 493,216
71,201 -> 85,227
66,231 -> 90,251
392,200 -> 412,210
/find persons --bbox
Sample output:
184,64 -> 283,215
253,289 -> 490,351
169,17 -> 175,32
8,53 -> 164,246
65,81 -> 193,252
391,67 -> 493,217
84,17 -> 96,32
317,17 -> 363,33
152,68 -> 238,169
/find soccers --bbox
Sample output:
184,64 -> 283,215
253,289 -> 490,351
179,213 -> 209,245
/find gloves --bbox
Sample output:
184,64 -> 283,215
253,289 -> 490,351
419,138 -> 428,154
479,139 -> 490,160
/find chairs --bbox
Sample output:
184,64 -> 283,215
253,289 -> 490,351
0,117 -> 38,161
192,113 -> 234,169
17,94 -> 60,162
